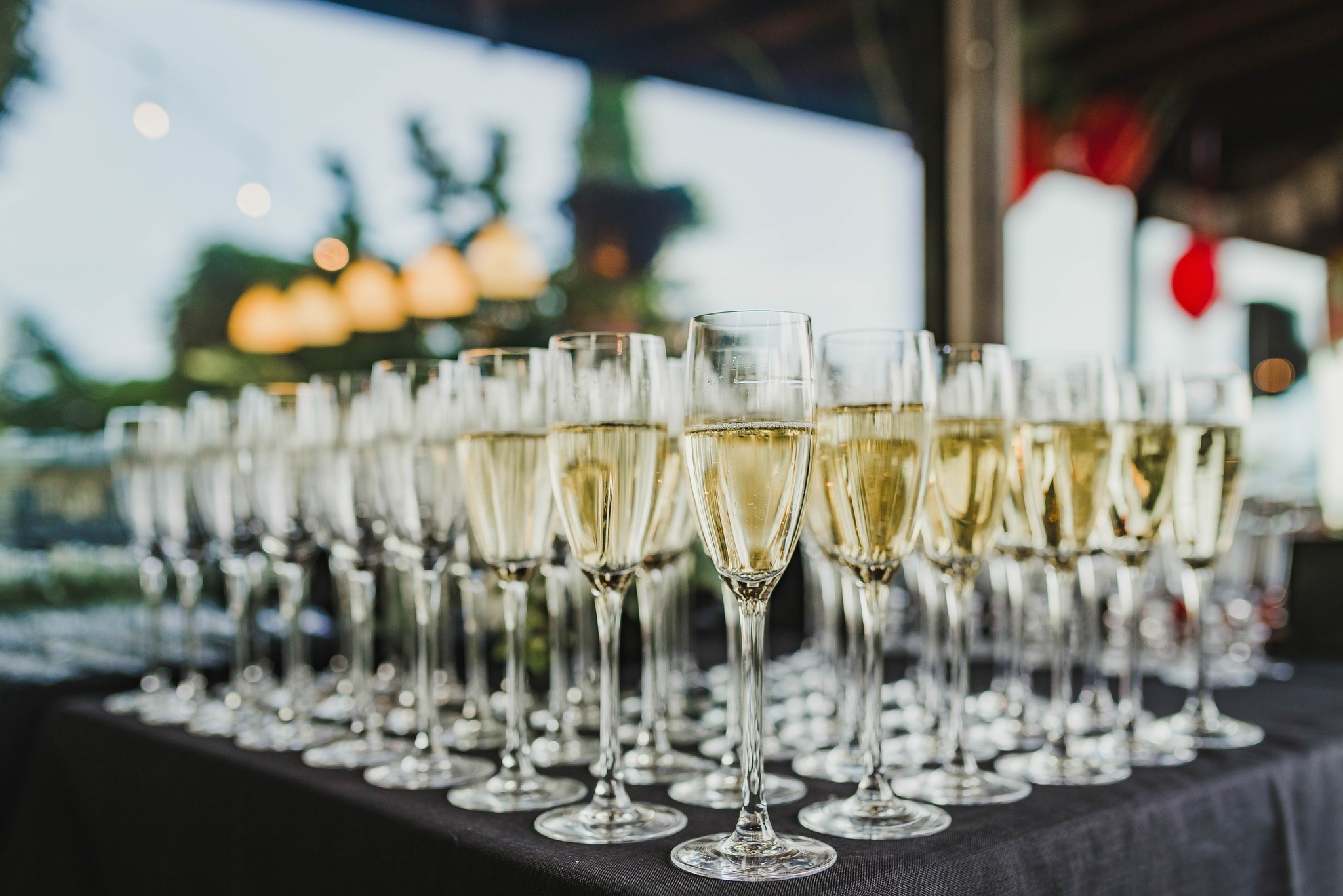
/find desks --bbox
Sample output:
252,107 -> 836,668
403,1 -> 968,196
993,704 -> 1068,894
0,631 -> 1343,896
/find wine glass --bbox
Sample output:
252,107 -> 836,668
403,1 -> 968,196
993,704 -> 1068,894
105,308 -> 1263,882
